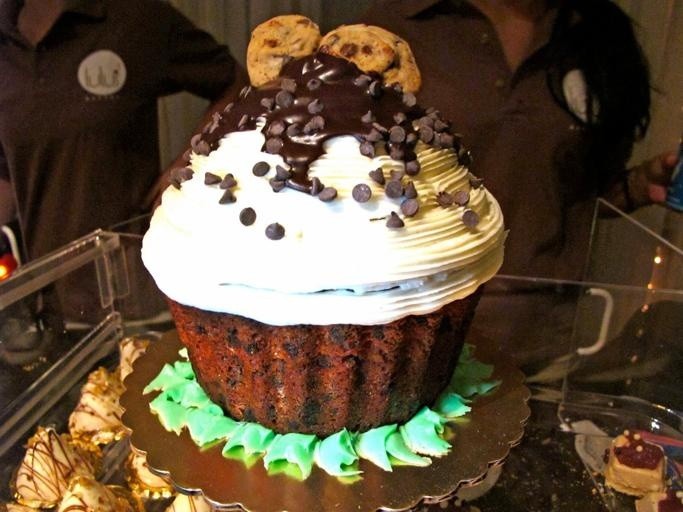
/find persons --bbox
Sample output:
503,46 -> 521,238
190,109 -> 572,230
0,1 -> 248,324
353,1 -> 682,379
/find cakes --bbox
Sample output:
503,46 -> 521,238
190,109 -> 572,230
124,451 -> 178,511
166,492 -> 215,512
633,491 -> 683,512
67,366 -> 129,442
7,425 -> 105,506
602,430 -> 673,499
139,13 -> 510,433
59,476 -> 139,512
117,336 -> 150,386
